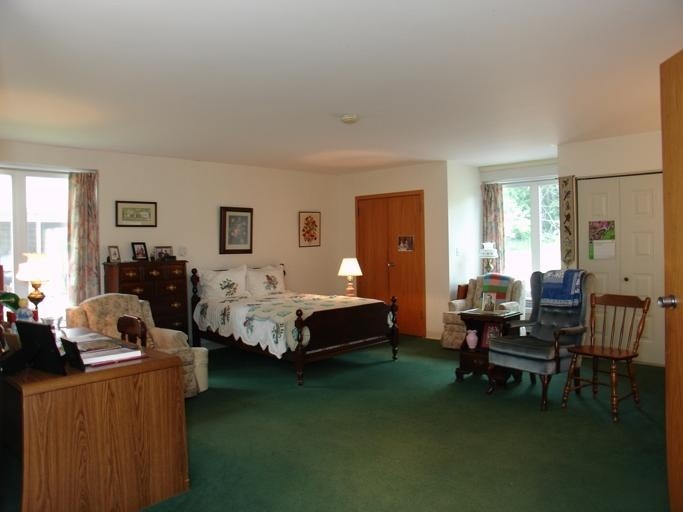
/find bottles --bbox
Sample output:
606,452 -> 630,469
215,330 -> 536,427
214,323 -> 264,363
466,329 -> 479,350
11,299 -> 32,337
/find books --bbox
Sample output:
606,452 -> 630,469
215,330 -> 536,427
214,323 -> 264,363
60,341 -> 151,367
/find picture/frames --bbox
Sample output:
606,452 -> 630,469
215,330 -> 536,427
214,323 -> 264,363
297,210 -> 321,247
152,244 -> 173,263
59,336 -> 87,373
105,245 -> 121,264
130,241 -> 148,261
12,318 -> 67,378
217,205 -> 253,255
114,200 -> 157,229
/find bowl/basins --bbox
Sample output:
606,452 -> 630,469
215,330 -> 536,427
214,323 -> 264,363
514,332 -> 530,338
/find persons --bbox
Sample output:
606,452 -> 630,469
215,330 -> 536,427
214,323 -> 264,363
483,295 -> 494,311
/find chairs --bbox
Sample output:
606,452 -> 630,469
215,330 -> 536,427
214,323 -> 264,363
63,290 -> 201,401
115,313 -> 148,350
437,264 -> 652,425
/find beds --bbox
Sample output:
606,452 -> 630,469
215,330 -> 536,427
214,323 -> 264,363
188,262 -> 401,388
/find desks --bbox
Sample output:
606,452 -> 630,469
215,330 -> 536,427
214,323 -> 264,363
0,324 -> 190,510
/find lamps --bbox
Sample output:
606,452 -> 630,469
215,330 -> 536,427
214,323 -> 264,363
479,240 -> 500,273
12,250 -> 63,324
335,257 -> 365,298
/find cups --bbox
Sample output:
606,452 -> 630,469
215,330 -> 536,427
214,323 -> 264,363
520,326 -> 527,336
40,317 -> 54,327
520,315 -> 526,320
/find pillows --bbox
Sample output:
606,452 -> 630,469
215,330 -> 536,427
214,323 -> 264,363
194,264 -> 251,306
241,264 -> 287,297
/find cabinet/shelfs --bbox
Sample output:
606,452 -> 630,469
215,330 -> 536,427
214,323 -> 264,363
99,258 -> 190,346
